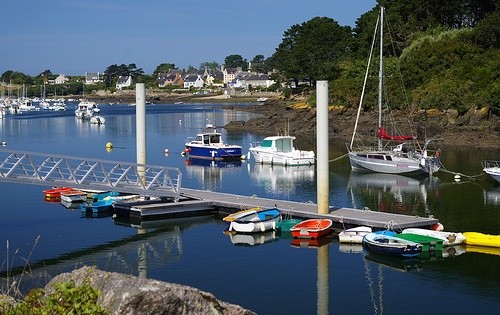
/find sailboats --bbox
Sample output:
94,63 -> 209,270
0,79 -> 88,115
345,7 -> 500,183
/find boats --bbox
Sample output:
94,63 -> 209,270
338,226 -> 500,259
223,229 -> 280,246
257,97 -> 268,102
181,124 -> 242,161
43,196 -> 115,218
290,233 -> 335,248
74,100 -> 105,124
289,219 -> 332,239
43,186 -> 162,213
223,207 -> 282,233
128,101 -> 151,107
338,242 -> 500,261
248,117 -> 315,166
